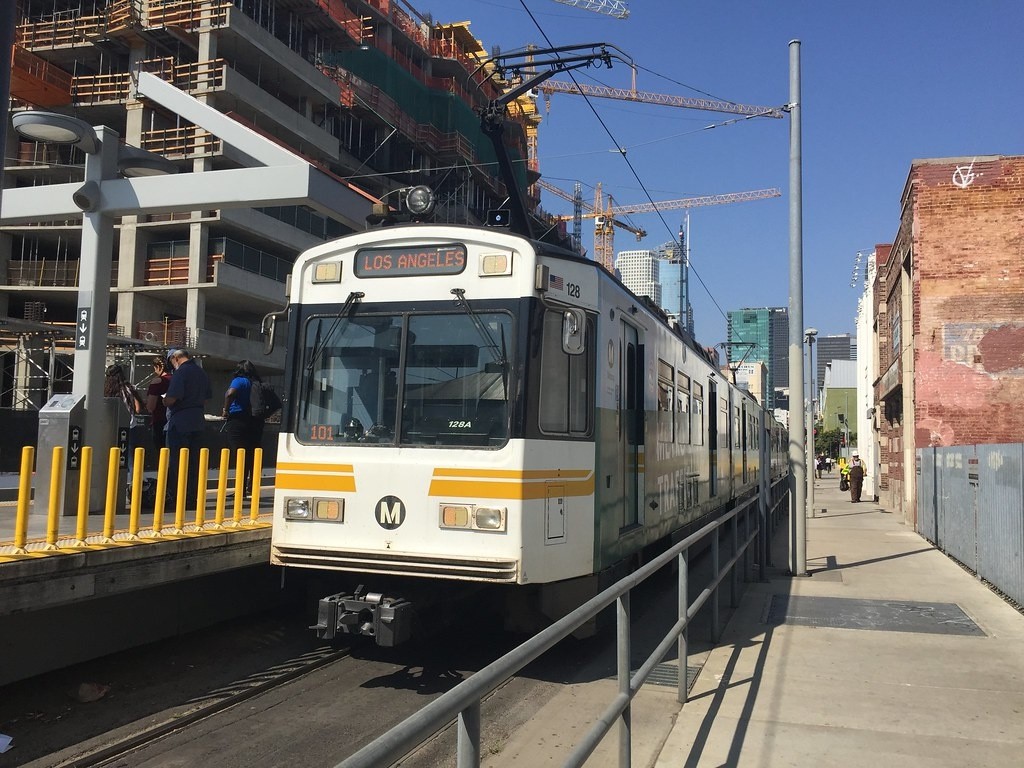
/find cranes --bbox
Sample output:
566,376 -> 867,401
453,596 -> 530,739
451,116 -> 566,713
544,182 -> 784,218
512,41 -> 784,171
534,176 -> 646,240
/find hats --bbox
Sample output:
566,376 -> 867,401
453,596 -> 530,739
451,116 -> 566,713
852,453 -> 859,457
166,349 -> 176,362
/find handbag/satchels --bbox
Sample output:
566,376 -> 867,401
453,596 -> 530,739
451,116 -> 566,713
840,479 -> 849,491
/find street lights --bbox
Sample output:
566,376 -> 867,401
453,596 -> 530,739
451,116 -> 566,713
807,326 -> 823,526
9,107 -> 182,517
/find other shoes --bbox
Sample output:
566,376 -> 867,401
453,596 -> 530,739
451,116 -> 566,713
246,491 -> 251,498
852,499 -> 860,503
226,492 -> 245,500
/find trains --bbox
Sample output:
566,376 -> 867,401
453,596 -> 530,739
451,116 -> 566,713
258,182 -> 788,646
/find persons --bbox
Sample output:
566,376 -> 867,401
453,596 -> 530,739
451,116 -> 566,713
221,359 -> 265,499
104,345 -> 212,511
814,450 -> 867,503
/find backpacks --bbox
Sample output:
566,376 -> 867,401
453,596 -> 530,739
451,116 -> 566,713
250,381 -> 281,419
